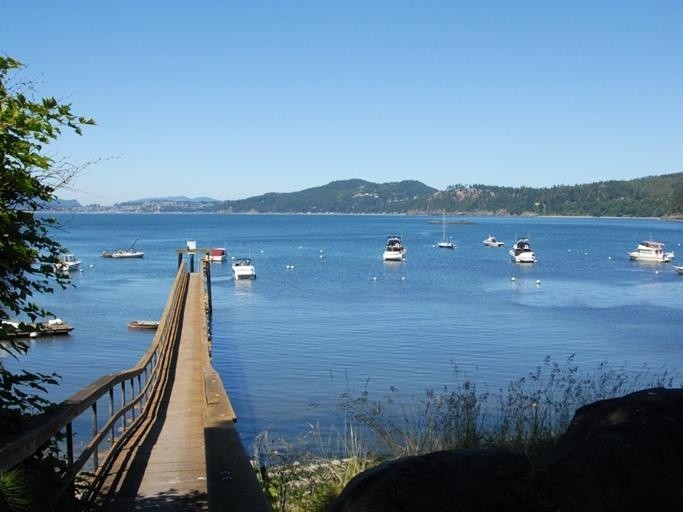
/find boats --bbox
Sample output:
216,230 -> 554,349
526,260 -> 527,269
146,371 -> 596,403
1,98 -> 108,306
508,232 -> 537,263
0,317 -> 74,338
125,319 -> 163,332
672,265 -> 682,275
42,252 -> 85,273
230,256 -> 256,280
625,239 -> 675,263
99,237 -> 148,260
482,232 -> 505,248
205,247 -> 230,263
382,234 -> 406,263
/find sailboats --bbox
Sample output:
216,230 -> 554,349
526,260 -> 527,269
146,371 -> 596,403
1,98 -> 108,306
436,208 -> 456,248
